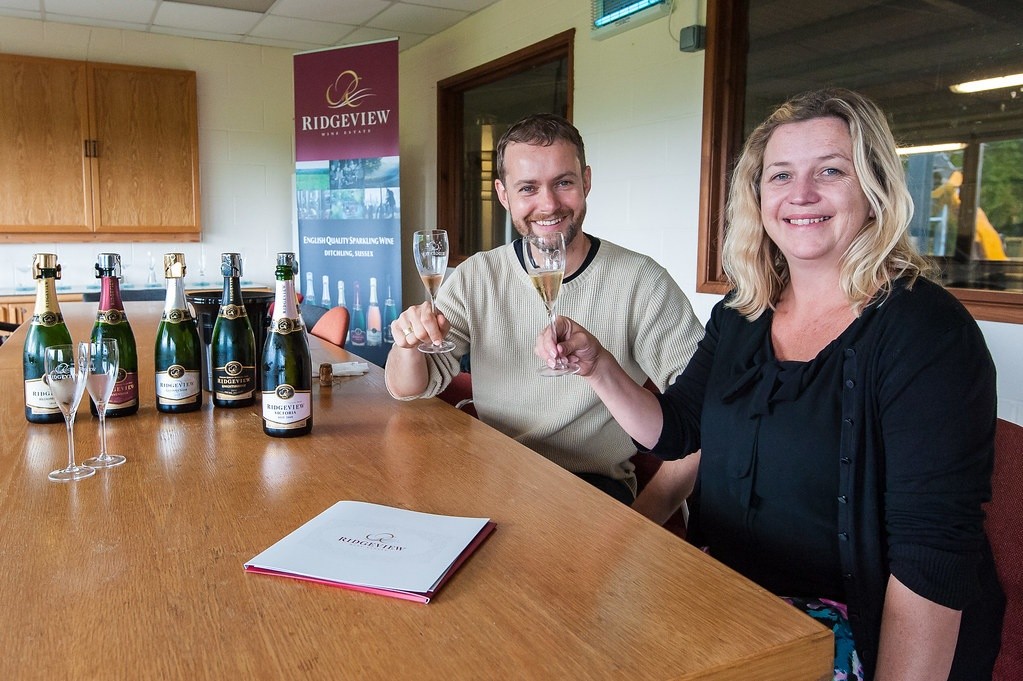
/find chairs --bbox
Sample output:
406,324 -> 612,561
446,368 -> 690,538
310,305 -> 350,347
986,417 -> 1023,681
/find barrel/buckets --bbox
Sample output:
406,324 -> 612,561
185,290 -> 276,393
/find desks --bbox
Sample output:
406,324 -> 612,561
1,298 -> 836,681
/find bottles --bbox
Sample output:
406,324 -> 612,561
349,281 -> 366,346
309,188 -> 321,221
89,253 -> 140,417
337,280 -> 350,345
297,188 -> 308,220
210,252 -> 257,409
382,275 -> 398,343
320,275 -> 332,311
322,186 -> 331,219
366,277 -> 382,348
155,252 -> 202,413
300,272 -> 317,307
261,253 -> 313,439
23,253 -> 76,424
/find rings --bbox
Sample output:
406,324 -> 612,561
404,326 -> 414,335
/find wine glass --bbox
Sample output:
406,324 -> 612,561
522,232 -> 581,378
412,229 -> 456,353
79,338 -> 127,468
44,344 -> 96,483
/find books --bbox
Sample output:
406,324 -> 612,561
244,500 -> 497,605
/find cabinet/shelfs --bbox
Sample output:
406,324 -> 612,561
0,53 -> 202,244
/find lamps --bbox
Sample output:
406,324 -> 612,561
589,0 -> 674,41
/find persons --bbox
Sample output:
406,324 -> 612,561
385,115 -> 707,533
535,90 -> 1004,681
